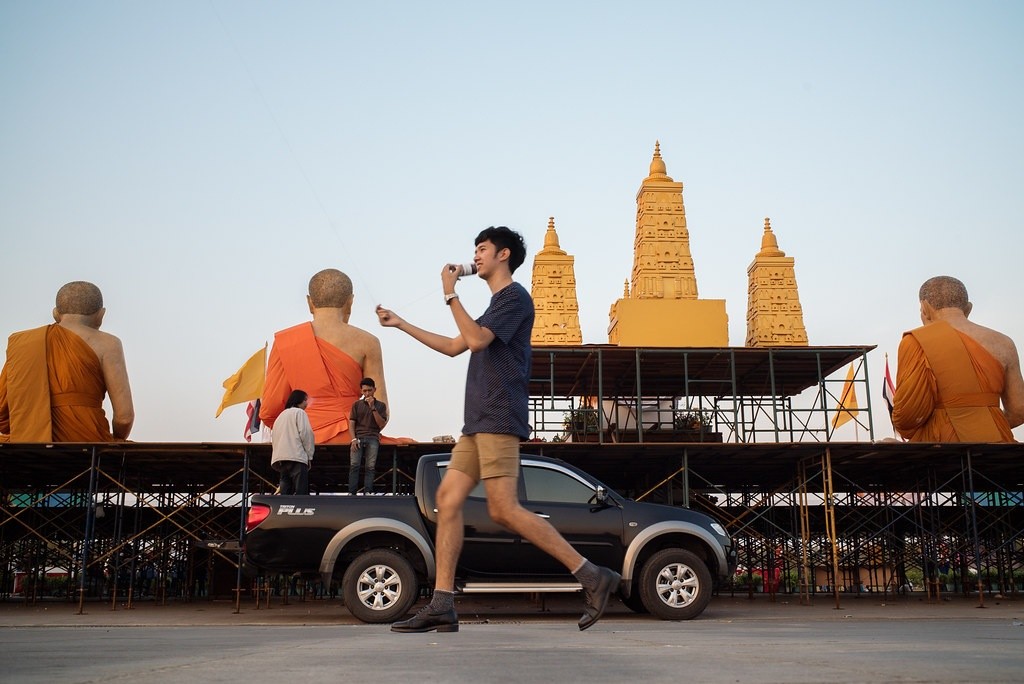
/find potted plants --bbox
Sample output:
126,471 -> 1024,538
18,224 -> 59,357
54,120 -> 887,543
669,406 -> 696,433
572,414 -> 585,431
22,575 -> 70,597
728,568 -> 814,594
587,415 -> 597,433
700,411 -> 713,433
938,567 -> 1024,592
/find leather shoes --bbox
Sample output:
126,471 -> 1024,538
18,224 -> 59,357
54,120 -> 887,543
390,605 -> 459,633
578,566 -> 622,631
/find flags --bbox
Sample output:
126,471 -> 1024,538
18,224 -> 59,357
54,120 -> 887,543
216,347 -> 268,442
829,357 -> 901,434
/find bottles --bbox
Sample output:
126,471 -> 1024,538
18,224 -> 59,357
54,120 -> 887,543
452,263 -> 478,276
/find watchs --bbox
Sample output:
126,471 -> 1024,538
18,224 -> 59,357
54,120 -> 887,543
372,407 -> 376,413
444,292 -> 457,304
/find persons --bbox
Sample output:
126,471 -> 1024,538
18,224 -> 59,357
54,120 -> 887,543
0,281 -> 135,442
376,225 -> 623,632
272,390 -> 315,494
880,276 -> 1024,445
347,377 -> 388,494
255,268 -> 418,443
737,560 -> 925,592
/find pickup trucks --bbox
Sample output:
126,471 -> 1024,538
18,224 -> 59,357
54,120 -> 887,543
242,451 -> 731,623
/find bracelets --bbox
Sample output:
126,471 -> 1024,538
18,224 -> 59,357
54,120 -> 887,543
350,437 -> 358,442
